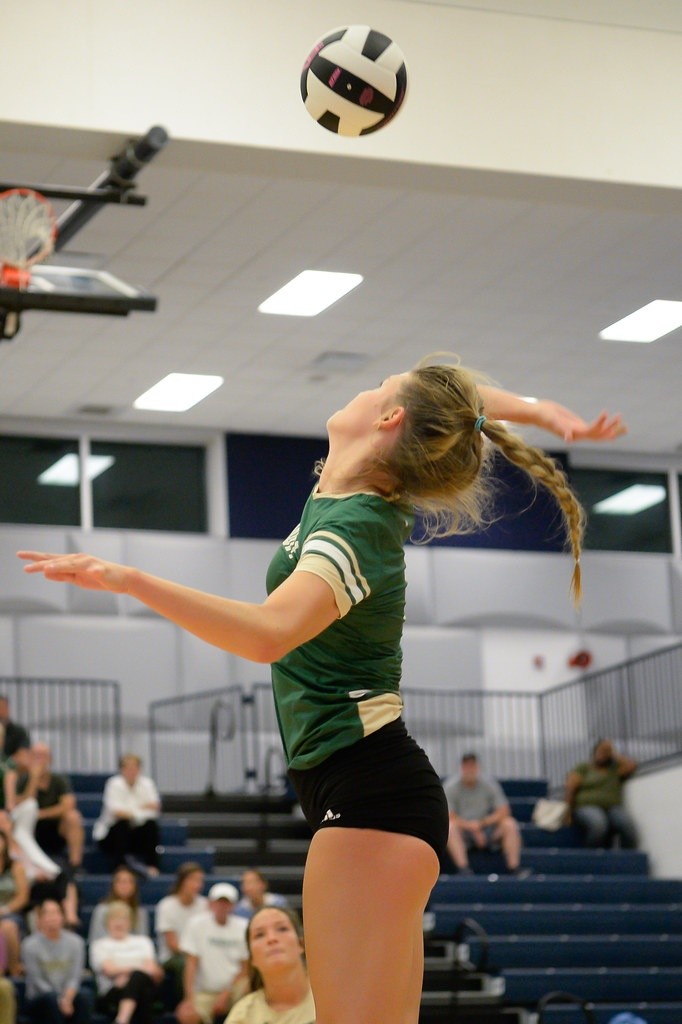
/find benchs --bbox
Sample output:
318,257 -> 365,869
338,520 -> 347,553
0,770 -> 250,1024
291,778 -> 682,1024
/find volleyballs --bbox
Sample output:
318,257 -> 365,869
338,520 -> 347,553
298,24 -> 410,140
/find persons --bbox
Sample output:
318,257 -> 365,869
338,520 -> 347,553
0,696 -> 316,1024
19,364 -> 625,1024
562,738 -> 640,850
441,751 -> 521,872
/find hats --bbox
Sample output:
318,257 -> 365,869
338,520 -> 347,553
462,753 -> 475,761
207,882 -> 239,903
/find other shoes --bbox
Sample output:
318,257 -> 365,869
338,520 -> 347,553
500,864 -> 532,876
